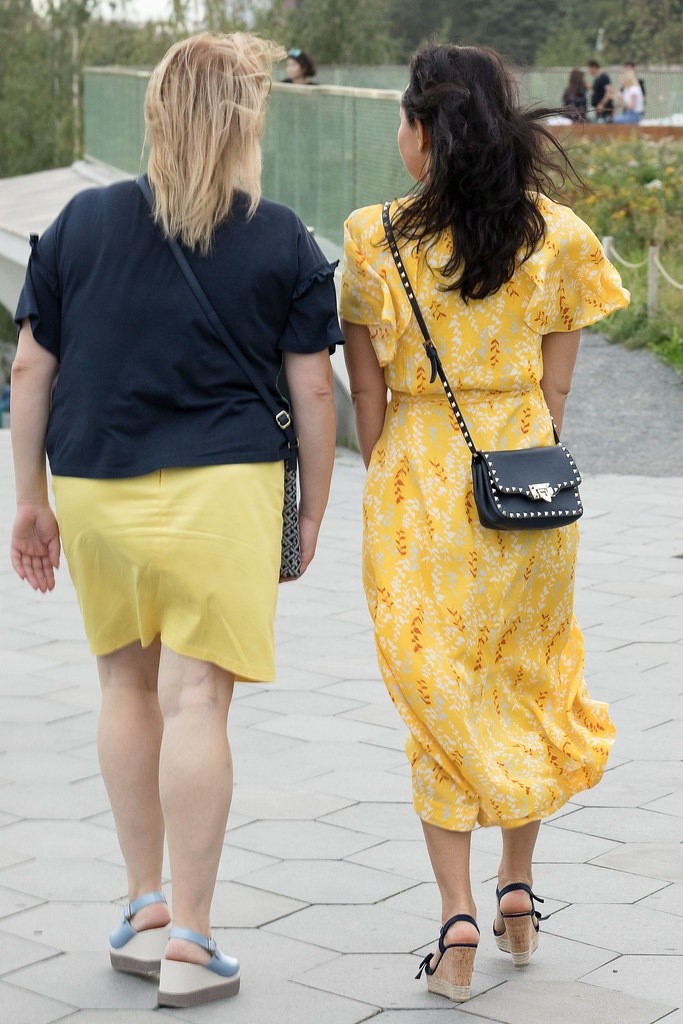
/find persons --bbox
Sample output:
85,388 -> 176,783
562,59 -> 646,126
280,47 -> 318,85
340,44 -> 630,1000
9,29 -> 345,1010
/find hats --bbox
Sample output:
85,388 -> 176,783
288,49 -> 314,77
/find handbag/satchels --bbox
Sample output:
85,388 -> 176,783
281,443 -> 302,578
473,444 -> 583,531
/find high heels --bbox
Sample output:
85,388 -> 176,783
110,893 -> 172,974
156,926 -> 241,1009
491,882 -> 544,965
415,914 -> 481,1001
